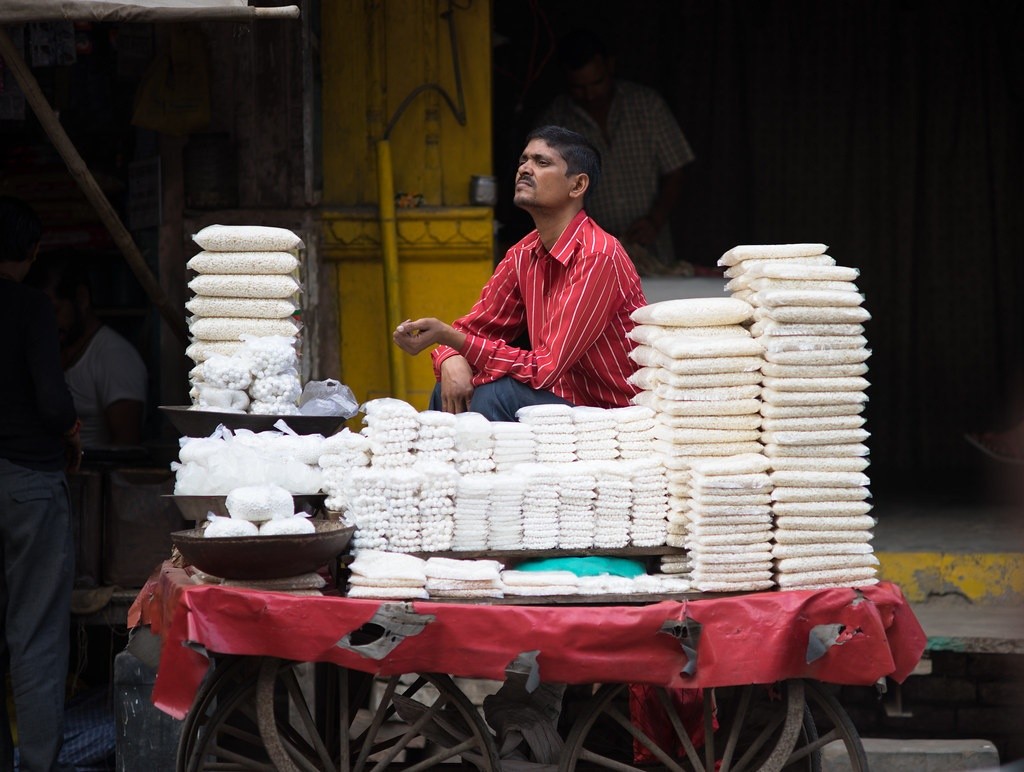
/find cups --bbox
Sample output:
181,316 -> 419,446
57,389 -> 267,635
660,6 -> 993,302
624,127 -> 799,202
470,176 -> 497,205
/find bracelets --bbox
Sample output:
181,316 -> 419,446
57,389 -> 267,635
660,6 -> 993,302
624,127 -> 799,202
67,421 -> 81,437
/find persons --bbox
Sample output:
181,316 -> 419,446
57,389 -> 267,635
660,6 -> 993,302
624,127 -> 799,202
25,252 -> 149,448
532,30 -> 695,263
394,124 -> 648,423
0,197 -> 83,772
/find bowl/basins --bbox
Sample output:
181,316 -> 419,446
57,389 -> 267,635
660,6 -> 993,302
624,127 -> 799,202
157,404 -> 346,440
173,520 -> 357,580
156,488 -> 328,526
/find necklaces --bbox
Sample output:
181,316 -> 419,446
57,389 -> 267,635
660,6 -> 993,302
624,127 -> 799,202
70,324 -> 103,361
